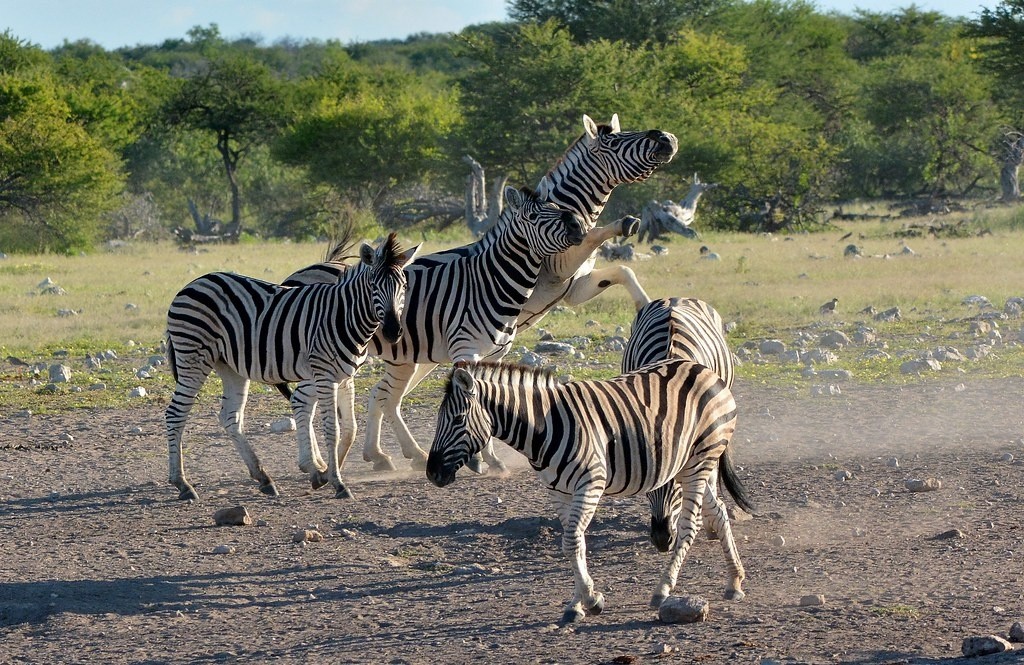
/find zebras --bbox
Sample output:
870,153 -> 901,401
426,298 -> 758,628
164,230 -> 423,503
276,111 -> 679,490
